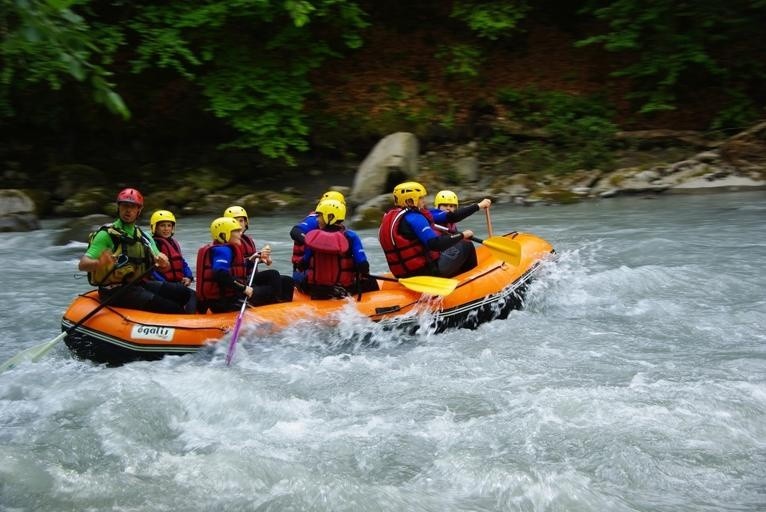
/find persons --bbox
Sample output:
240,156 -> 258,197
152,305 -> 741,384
79,187 -> 190,314
150,210 -> 197,314
379,182 -> 478,278
225,206 -> 294,304
197,216 -> 279,314
294,198 -> 380,297
289,191 -> 348,286
432,188 -> 492,237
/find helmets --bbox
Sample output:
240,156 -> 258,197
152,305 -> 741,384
392,181 -> 426,209
149,209 -> 176,234
116,188 -> 144,208
223,206 -> 248,228
433,190 -> 458,213
315,200 -> 345,226
209,216 -> 242,244
319,191 -> 346,207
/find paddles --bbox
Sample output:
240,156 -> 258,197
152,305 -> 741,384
226,257 -> 263,368
360,273 -> 460,296
433,220 -> 522,266
0,268 -> 161,372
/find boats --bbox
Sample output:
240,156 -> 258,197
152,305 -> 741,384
60,231 -> 559,367
